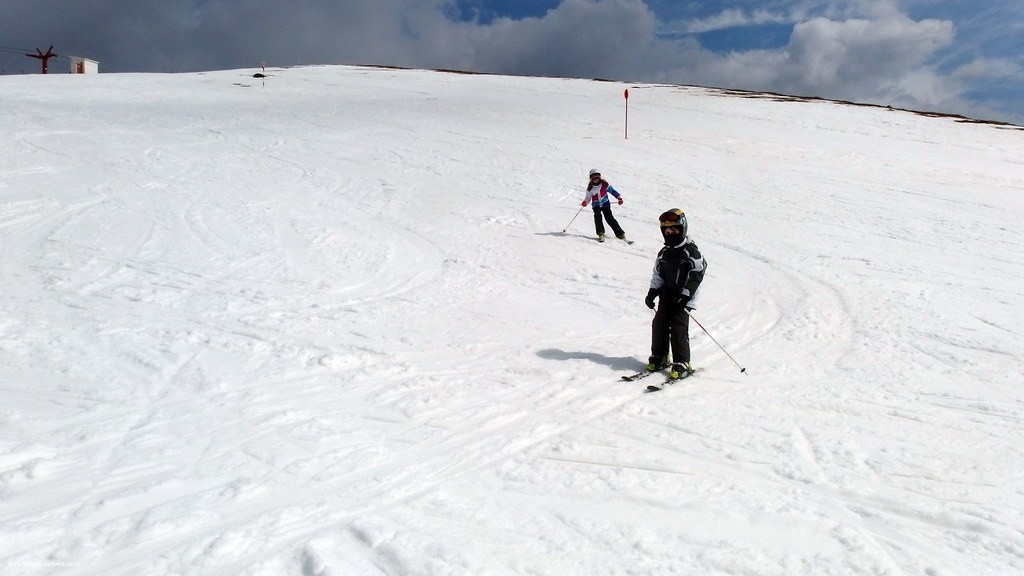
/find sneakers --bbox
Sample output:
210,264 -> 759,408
669,361 -> 692,380
646,353 -> 670,371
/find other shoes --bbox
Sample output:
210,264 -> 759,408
621,236 -> 627,241
599,232 -> 604,242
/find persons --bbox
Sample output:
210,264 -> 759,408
582,168 -> 626,242
645,208 -> 707,381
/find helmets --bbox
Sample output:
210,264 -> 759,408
659,208 -> 688,239
589,168 -> 601,179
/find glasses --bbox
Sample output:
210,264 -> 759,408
659,212 -> 679,223
591,176 -> 600,181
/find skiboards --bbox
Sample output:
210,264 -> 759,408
621,364 -> 705,390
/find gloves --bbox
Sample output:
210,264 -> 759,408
645,288 -> 658,309
618,196 -> 623,205
673,296 -> 685,315
582,200 -> 589,207
